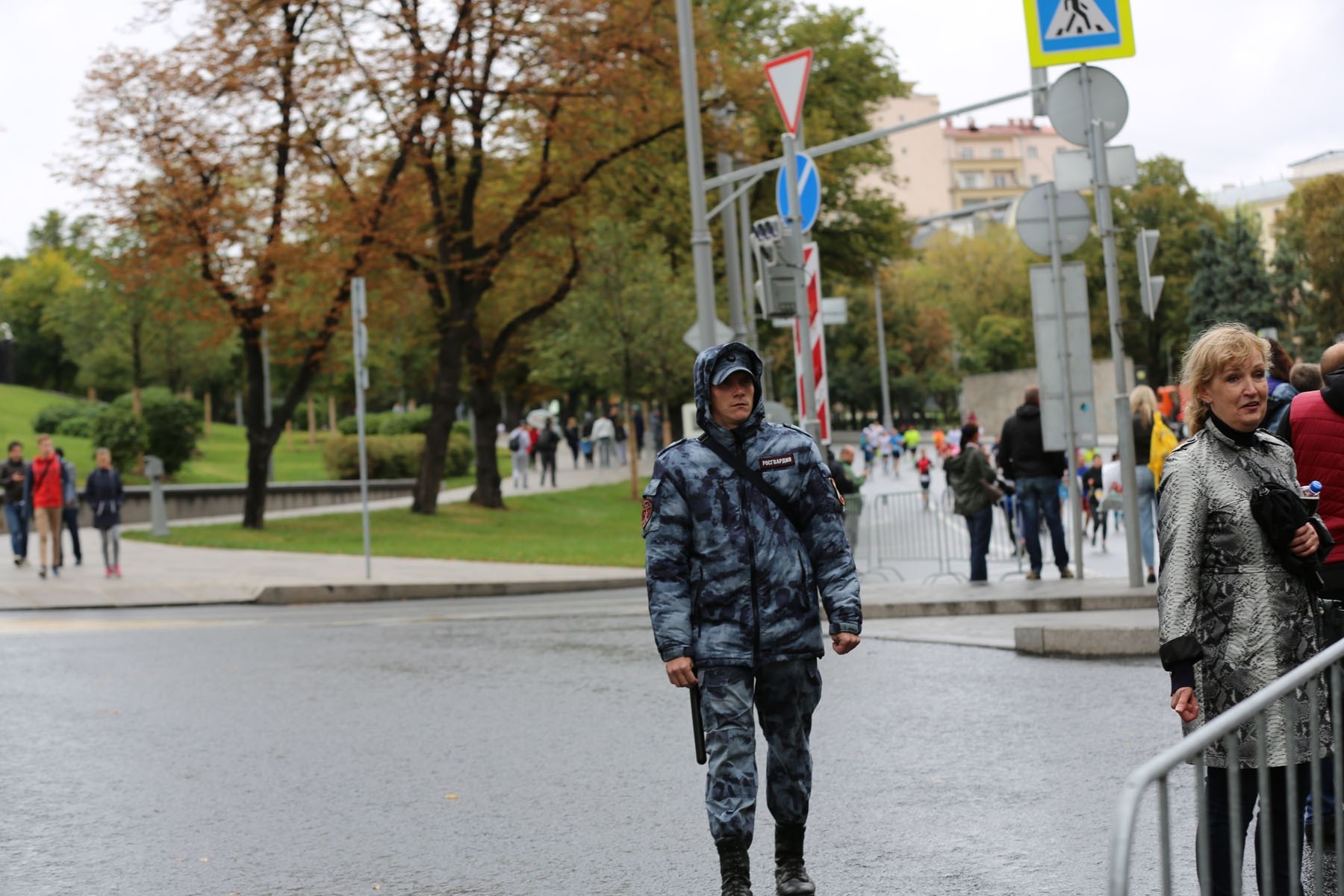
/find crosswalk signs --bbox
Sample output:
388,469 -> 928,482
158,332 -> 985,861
1023,1 -> 1138,67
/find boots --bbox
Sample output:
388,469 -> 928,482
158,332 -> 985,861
716,838 -> 754,896
774,821 -> 816,896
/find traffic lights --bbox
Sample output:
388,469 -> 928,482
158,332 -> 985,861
750,216 -> 783,243
1133,228 -> 1167,321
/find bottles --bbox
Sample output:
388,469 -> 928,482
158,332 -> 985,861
1301,480 -> 1323,498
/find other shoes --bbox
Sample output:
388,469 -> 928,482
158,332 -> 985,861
1147,574 -> 1156,583
1060,567 -> 1075,579
1026,570 -> 1041,580
14,554 -> 23,564
39,572 -> 47,579
53,566 -> 61,579
113,566 -> 122,577
104,567 -> 113,578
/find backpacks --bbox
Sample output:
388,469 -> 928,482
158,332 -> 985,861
1147,411 -> 1179,494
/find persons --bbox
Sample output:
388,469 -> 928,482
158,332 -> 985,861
85,447 -> 126,580
992,434 -> 1124,558
648,409 -> 663,453
826,445 -> 867,555
913,445 -> 935,511
1113,382 -> 1180,583
1000,389 -> 1075,578
642,339 -> 863,896
860,421 -> 962,478
1157,333 -> 1344,838
505,397 -> 645,488
943,420 -> 1002,581
0,436 -> 82,578
1155,320 -> 1336,896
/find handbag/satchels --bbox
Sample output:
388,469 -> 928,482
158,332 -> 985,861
509,434 -> 520,452
1317,597 -> 1344,653
1096,459 -> 1124,512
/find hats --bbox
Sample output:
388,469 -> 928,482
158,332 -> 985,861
711,351 -> 755,386
